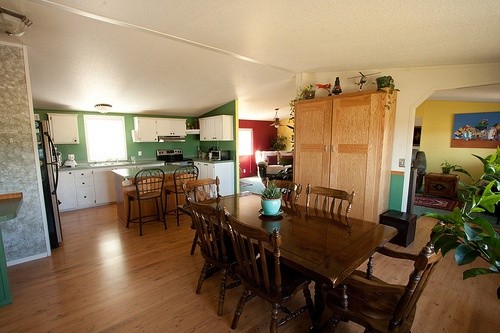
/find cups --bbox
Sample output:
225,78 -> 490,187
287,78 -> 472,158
130,156 -> 136,163
68,154 -> 75,160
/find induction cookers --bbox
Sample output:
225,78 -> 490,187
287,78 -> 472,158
157,148 -> 192,162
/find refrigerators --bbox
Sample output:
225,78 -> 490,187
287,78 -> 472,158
35,120 -> 63,249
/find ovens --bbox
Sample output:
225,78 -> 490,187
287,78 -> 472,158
166,161 -> 194,167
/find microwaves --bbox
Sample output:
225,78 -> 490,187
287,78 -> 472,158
211,150 -> 230,161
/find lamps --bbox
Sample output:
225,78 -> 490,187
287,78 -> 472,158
94,104 -> 112,113
269,108 -> 284,128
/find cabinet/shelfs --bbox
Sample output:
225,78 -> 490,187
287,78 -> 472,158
132,116 -> 187,142
198,114 -> 233,142
424,172 -> 458,199
53,167 -> 119,211
194,161 -> 234,197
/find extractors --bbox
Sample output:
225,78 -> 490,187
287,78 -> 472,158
157,136 -> 186,142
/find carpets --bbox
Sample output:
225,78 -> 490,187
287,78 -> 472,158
414,194 -> 463,211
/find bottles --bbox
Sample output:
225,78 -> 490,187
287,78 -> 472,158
331,77 -> 342,94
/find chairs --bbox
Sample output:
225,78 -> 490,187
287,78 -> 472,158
318,241 -> 446,333
125,168 -> 167,237
164,164 -> 199,227
186,176 -> 355,333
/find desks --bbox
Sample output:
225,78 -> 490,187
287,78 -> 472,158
177,190 -> 398,333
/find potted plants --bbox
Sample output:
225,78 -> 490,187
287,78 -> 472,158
261,180 -> 283,216
440,158 -> 457,174
290,83 -> 316,115
